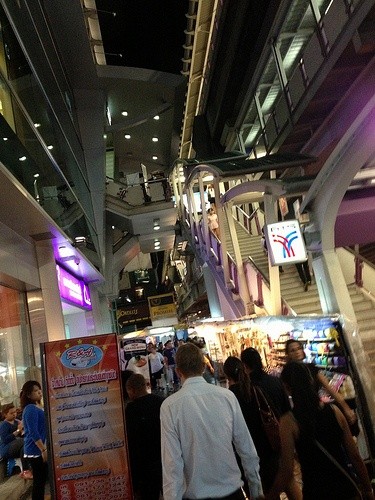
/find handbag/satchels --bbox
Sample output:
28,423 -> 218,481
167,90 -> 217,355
253,385 -> 281,449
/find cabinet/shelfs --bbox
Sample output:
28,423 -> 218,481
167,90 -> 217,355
271,334 -> 347,374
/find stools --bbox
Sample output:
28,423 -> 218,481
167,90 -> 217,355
6,458 -> 23,477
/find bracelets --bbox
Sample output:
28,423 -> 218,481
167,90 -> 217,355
16,429 -> 20,434
41,448 -> 47,453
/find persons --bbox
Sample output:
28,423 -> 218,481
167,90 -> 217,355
241,347 -> 299,445
208,208 -> 221,242
20,380 -> 49,500
159,343 -> 265,500
207,185 -> 216,213
223,355 -> 301,500
261,225 -> 284,273
267,359 -> 375,500
278,338 -> 357,426
121,373 -> 164,500
148,336 -> 218,397
295,260 -> 312,291
0,404 -> 34,480
